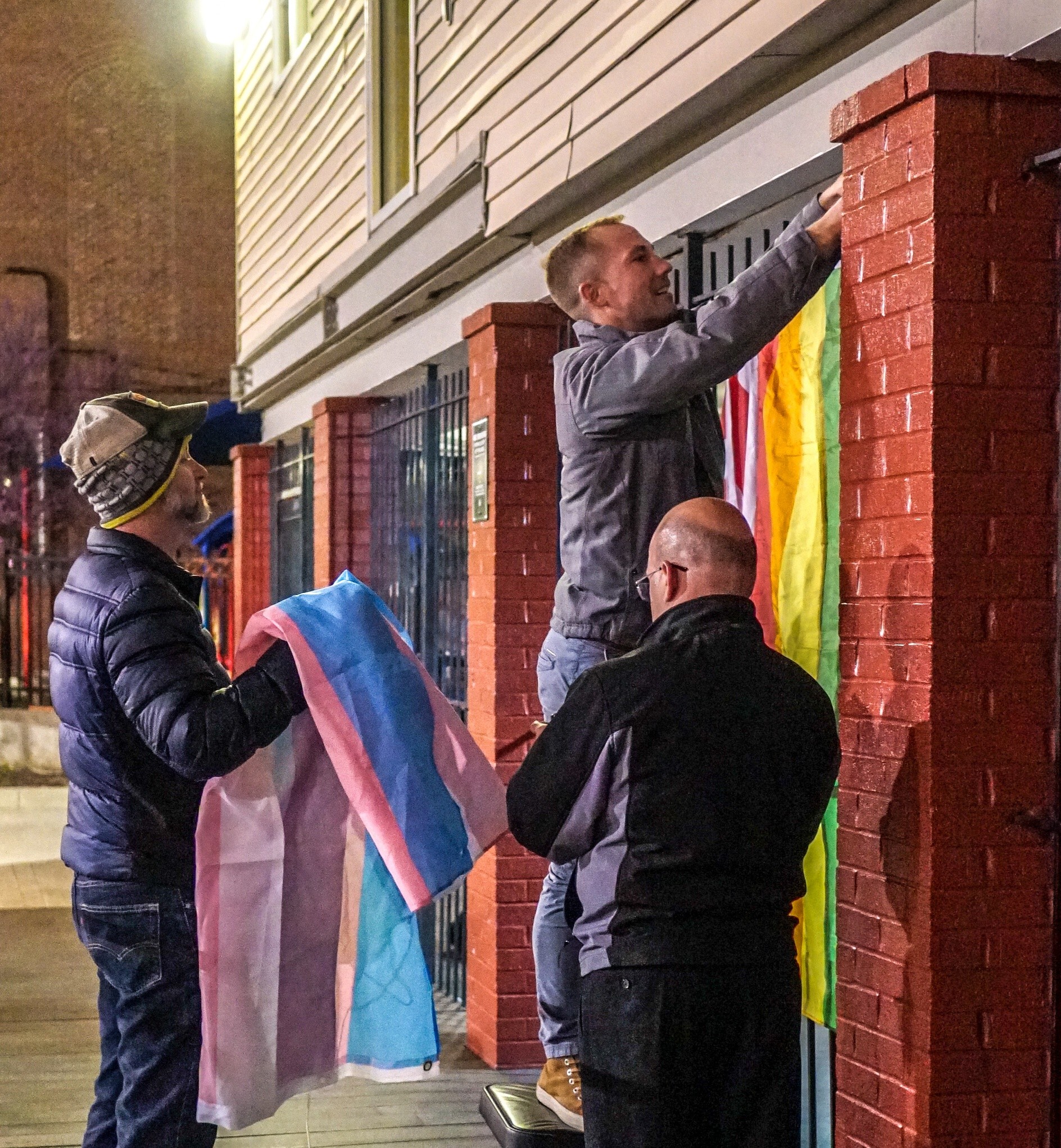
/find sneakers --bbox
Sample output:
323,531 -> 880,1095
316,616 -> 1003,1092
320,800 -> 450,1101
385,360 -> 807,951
536,1056 -> 584,1134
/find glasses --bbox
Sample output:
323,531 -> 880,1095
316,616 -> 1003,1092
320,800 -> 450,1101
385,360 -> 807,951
634,563 -> 688,602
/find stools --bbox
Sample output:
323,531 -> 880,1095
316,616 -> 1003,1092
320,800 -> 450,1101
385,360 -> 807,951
478,1081 -> 586,1148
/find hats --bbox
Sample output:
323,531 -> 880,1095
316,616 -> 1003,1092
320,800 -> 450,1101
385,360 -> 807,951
59,391 -> 207,529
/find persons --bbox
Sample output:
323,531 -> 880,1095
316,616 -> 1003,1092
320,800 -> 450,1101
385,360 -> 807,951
505,497 -> 841,1148
47,393 -> 308,1148
530,176 -> 843,1133
